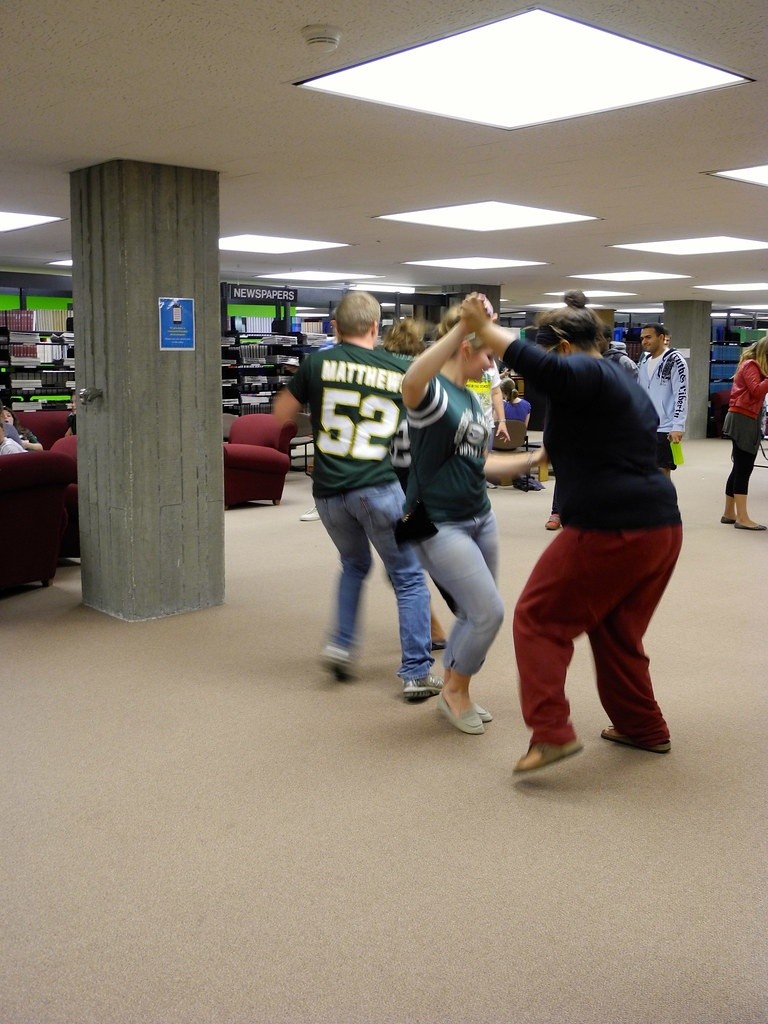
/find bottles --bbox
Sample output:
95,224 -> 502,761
670,441 -> 684,465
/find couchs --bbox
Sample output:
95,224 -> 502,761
48,434 -> 81,560
0,449 -> 76,592
223,413 -> 300,508
10,410 -> 79,452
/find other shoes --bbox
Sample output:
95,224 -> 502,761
734,522 -> 766,531
474,704 -> 493,722
300,507 -> 322,521
545,516 -> 561,530
513,742 -> 584,777
403,674 -> 443,698
432,641 -> 448,651
720,516 -> 738,524
602,726 -> 671,753
486,482 -> 497,490
317,643 -> 356,683
437,693 -> 485,735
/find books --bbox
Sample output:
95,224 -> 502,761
220,315 -> 333,411
0,307 -> 77,391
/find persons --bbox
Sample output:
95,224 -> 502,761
720,336 -> 767,531
65,392 -> 77,437
275,291 -> 547,736
599,322 -> 690,480
458,291 -> 684,773
0,406 -> 44,455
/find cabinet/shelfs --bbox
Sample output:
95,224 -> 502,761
1,322 -> 77,408
221,329 -> 315,410
706,339 -> 745,440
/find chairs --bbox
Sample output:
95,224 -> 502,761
291,413 -> 319,472
491,419 -> 531,492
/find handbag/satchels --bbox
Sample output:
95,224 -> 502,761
394,501 -> 439,548
